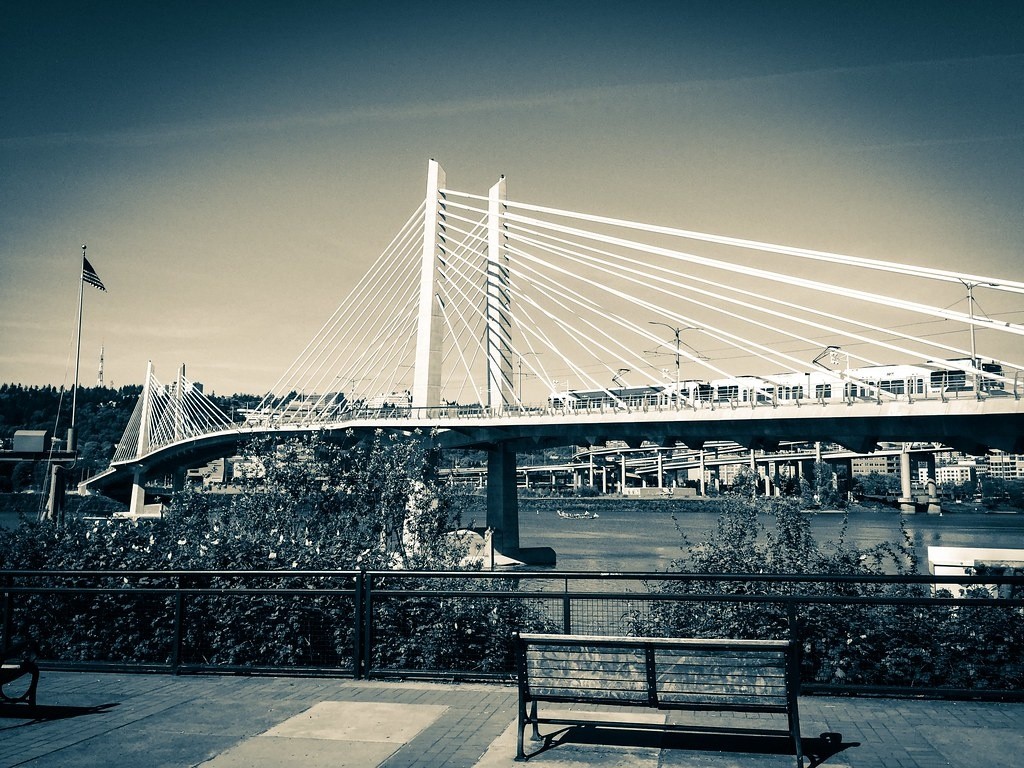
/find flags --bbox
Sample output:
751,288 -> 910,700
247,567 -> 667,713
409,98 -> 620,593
82,257 -> 107,294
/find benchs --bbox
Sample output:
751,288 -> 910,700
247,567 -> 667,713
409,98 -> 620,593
512,632 -> 803,768
0,637 -> 39,717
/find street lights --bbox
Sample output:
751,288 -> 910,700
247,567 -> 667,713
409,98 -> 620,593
518,351 -> 539,416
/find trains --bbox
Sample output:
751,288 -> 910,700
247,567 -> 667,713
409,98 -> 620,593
547,345 -> 1006,410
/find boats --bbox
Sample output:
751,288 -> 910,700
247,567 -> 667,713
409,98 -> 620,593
556,509 -> 599,519
